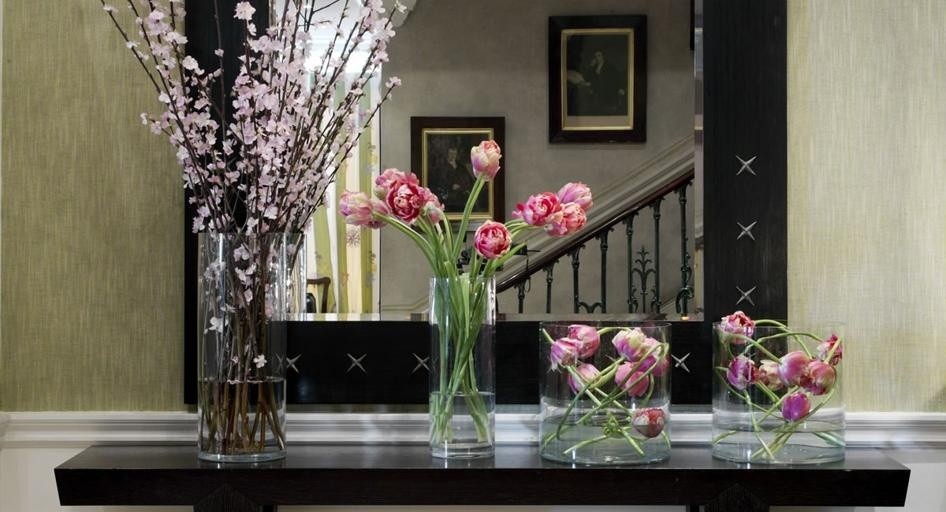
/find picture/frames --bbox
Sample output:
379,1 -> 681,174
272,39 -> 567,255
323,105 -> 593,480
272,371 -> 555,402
405,111 -> 509,242
542,10 -> 652,148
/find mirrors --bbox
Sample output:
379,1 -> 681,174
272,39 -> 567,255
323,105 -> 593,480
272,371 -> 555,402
174,0 -> 795,413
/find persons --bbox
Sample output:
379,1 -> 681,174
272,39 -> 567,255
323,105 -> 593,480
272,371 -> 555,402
428,134 -> 477,212
584,48 -> 626,110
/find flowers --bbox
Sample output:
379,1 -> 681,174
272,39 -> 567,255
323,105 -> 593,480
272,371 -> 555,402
97,0 -> 406,452
336,132 -> 590,438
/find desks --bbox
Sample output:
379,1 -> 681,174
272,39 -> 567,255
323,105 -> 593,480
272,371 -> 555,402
52,438 -> 912,512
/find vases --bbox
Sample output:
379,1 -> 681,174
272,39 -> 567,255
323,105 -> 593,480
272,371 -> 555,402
423,271 -> 499,461
190,228 -> 293,467
